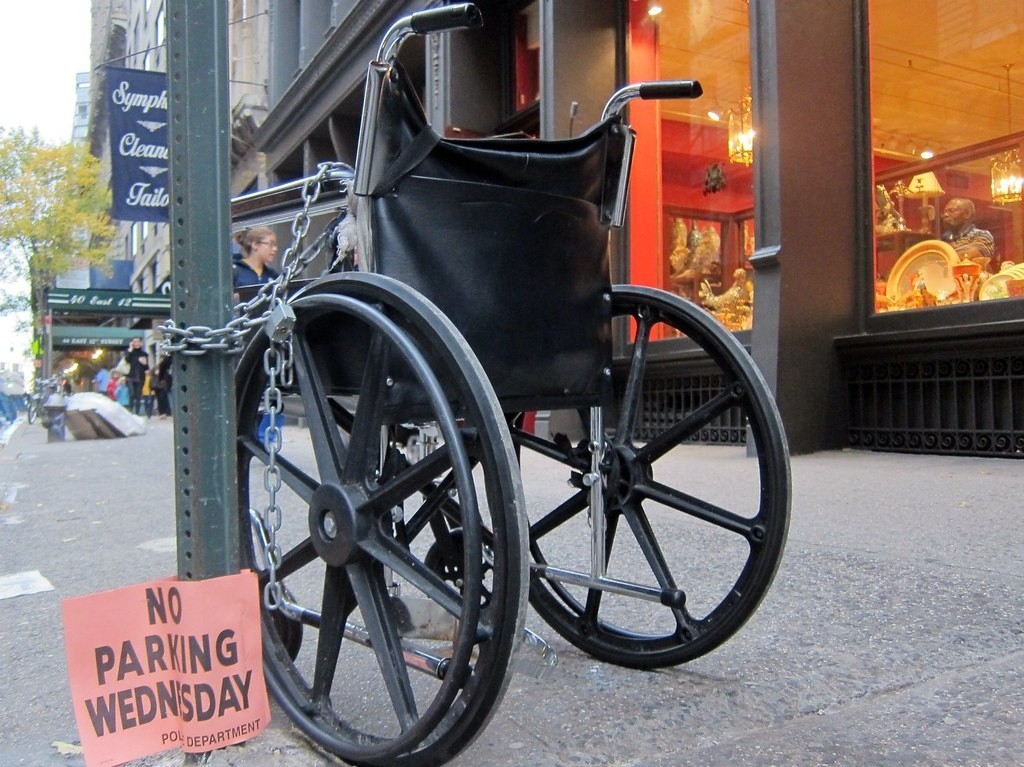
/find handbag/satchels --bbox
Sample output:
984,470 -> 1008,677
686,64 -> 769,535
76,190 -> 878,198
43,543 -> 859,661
148,375 -> 168,390
117,356 -> 131,375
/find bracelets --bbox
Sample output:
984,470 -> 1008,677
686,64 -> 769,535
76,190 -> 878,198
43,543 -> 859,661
961,249 -> 969,259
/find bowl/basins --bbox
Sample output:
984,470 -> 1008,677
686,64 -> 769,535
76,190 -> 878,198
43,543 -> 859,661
952,253 -> 982,281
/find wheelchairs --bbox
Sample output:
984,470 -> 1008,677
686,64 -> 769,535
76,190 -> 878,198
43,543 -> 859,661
238,1 -> 793,767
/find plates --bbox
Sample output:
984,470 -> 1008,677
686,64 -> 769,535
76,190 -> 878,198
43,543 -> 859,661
884,239 -> 958,306
979,262 -> 1024,302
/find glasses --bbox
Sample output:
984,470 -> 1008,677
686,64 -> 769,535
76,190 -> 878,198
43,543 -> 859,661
256,240 -> 279,249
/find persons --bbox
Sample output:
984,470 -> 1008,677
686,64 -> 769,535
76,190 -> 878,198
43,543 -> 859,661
231,228 -> 283,371
961,273 -> 971,296
96,365 -> 130,408
941,197 -> 995,262
914,272 -> 926,308
126,337 -> 173,421
62,377 -> 71,396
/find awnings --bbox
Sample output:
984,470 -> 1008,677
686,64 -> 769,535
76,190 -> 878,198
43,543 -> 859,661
46,289 -> 172,319
51,326 -> 144,351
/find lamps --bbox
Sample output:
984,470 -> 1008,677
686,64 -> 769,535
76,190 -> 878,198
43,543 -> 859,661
990,62 -> 1022,203
903,171 -> 945,234
727,83 -> 753,169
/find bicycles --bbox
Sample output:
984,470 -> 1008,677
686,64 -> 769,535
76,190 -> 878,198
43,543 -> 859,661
28,378 -> 60,424
